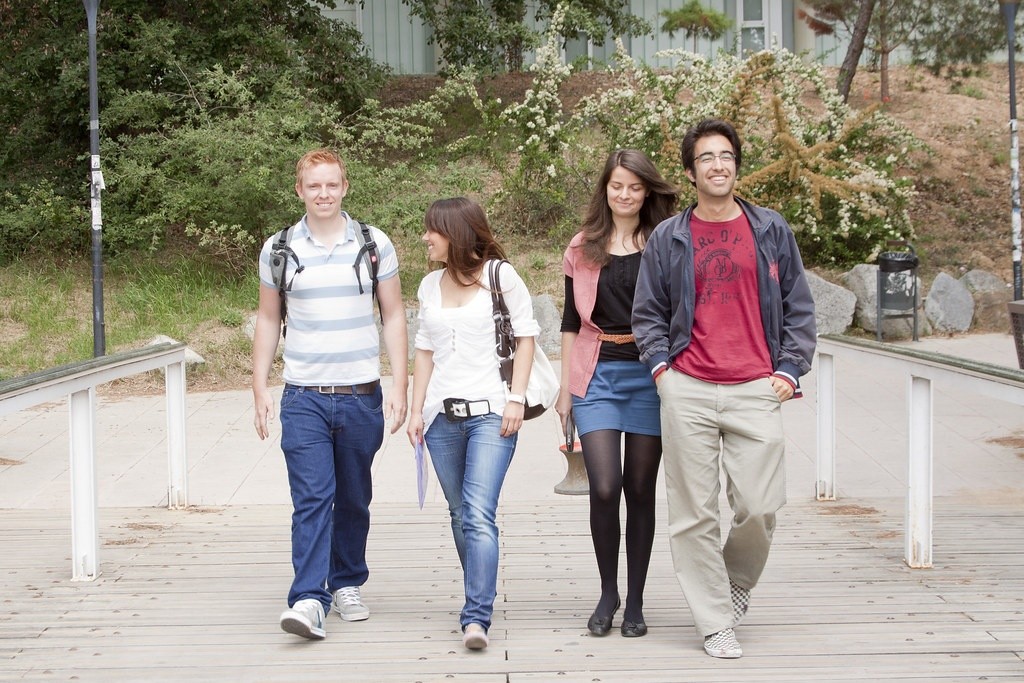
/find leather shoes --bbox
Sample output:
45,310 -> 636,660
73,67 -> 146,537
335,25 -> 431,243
588,596 -> 620,634
621,619 -> 648,636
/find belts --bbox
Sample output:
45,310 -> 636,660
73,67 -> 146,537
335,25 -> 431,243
285,379 -> 380,394
439,398 -> 490,422
598,334 -> 635,344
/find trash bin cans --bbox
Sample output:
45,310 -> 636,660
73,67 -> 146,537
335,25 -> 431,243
876,249 -> 919,310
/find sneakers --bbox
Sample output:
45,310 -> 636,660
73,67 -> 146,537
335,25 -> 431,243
704,628 -> 742,658
280,599 -> 327,639
331,586 -> 369,622
728,577 -> 751,628
464,622 -> 489,648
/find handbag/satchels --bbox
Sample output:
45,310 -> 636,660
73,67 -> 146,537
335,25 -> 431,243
489,258 -> 560,420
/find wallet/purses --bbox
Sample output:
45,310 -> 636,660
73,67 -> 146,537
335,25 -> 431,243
566,413 -> 575,453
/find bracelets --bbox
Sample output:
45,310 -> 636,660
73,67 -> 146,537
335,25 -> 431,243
505,393 -> 526,405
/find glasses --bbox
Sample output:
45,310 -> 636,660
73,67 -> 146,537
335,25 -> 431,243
693,154 -> 736,164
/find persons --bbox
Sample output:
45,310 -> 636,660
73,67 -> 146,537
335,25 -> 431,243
252,152 -> 408,640
404,197 -> 544,652
555,149 -> 681,638
632,119 -> 818,662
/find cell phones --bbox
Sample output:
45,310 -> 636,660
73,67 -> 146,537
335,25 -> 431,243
567,413 -> 576,451
